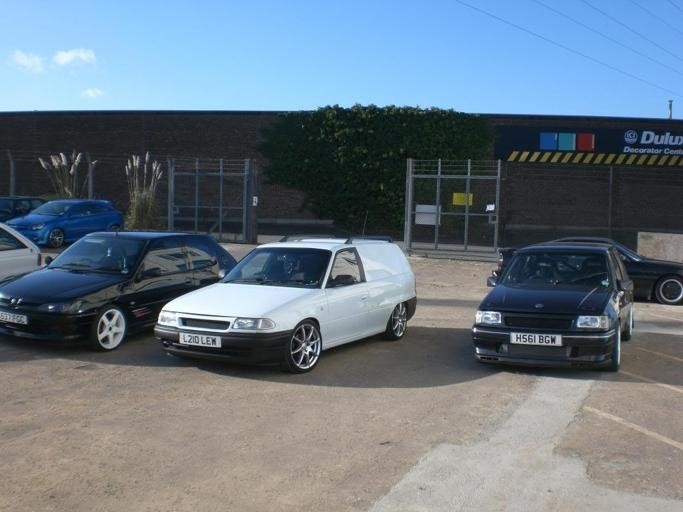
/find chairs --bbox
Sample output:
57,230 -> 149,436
575,258 -> 603,287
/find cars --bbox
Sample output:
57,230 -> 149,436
0,220 -> 41,283
7,199 -> 122,248
0,194 -> 47,220
470,244 -> 631,375
487,236 -> 683,307
0,231 -> 241,352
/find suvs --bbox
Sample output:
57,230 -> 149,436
154,235 -> 419,373
